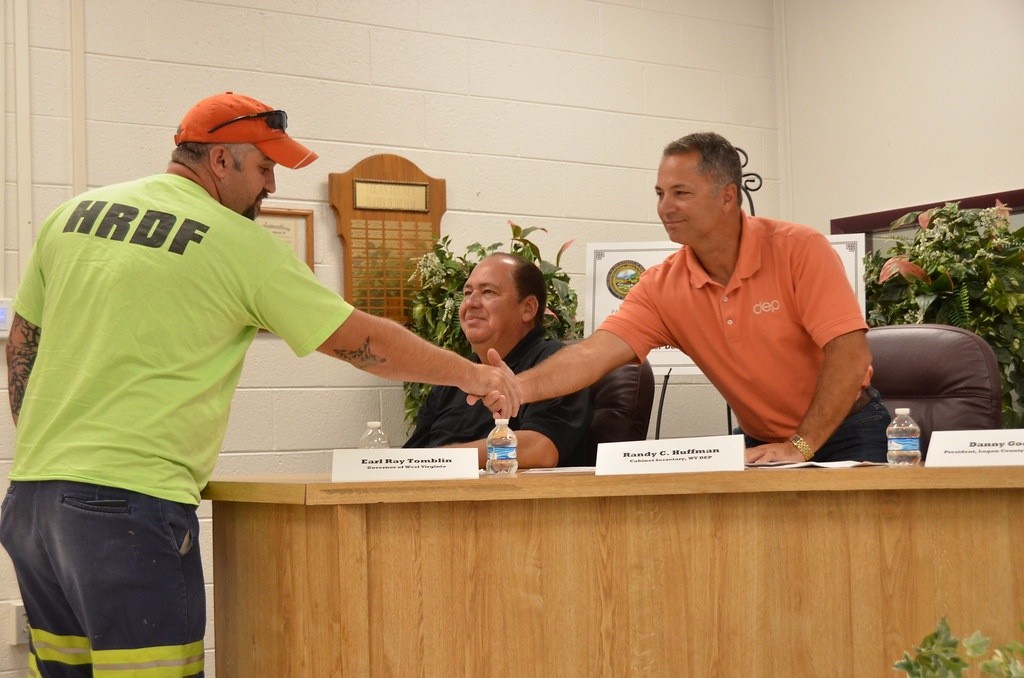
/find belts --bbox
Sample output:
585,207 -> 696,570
844,386 -> 879,416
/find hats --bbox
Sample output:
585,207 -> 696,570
174,91 -> 319,170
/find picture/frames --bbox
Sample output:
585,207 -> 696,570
254,206 -> 314,333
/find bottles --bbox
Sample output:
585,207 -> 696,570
487,419 -> 518,475
360,422 -> 390,449
886,408 -> 921,467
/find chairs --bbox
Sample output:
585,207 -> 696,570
559,340 -> 656,467
862,323 -> 1002,460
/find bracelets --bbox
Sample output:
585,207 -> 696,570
789,434 -> 814,460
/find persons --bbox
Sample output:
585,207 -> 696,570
402,252 -> 588,469
470,131 -> 893,465
0,92 -> 523,678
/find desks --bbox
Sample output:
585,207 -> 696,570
200,462 -> 1024,677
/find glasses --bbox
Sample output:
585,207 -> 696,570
208,109 -> 288,134
